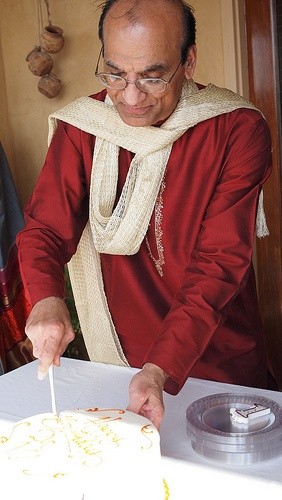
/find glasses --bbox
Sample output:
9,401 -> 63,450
96,47 -> 185,95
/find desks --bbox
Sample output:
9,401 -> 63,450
0,354 -> 282,500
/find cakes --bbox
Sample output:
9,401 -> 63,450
0,407 -> 183,500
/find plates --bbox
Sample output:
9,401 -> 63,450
184,392 -> 282,445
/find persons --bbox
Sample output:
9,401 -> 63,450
3,2 -> 277,431
